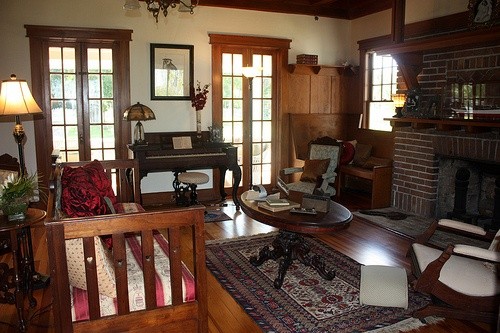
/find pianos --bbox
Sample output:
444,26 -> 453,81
126,131 -> 241,210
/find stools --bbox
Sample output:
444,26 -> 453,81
178,172 -> 208,217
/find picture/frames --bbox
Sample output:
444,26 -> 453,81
401,87 -> 423,116
150,43 -> 194,101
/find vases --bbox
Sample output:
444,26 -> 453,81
196,110 -> 203,143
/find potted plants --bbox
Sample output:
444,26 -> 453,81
0,169 -> 49,221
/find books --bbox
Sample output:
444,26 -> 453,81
257,198 -> 300,212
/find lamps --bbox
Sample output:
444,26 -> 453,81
239,66 -> 264,90
122,102 -> 156,144
122,0 -> 199,23
0,74 -> 50,290
391,94 -> 407,117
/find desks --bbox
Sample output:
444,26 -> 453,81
0,208 -> 46,333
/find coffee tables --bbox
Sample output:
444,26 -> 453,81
240,190 -> 353,288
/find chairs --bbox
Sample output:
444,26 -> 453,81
405,219 -> 500,333
0,153 -> 24,257
277,137 -> 344,200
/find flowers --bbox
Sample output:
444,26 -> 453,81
190,81 -> 211,112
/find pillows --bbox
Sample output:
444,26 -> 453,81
360,264 -> 408,310
289,126 -> 374,195
60,160 -> 124,298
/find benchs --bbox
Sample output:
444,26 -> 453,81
44,150 -> 208,333
290,112 -> 393,209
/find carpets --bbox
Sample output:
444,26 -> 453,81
205,230 -> 445,333
206,210 -> 231,223
